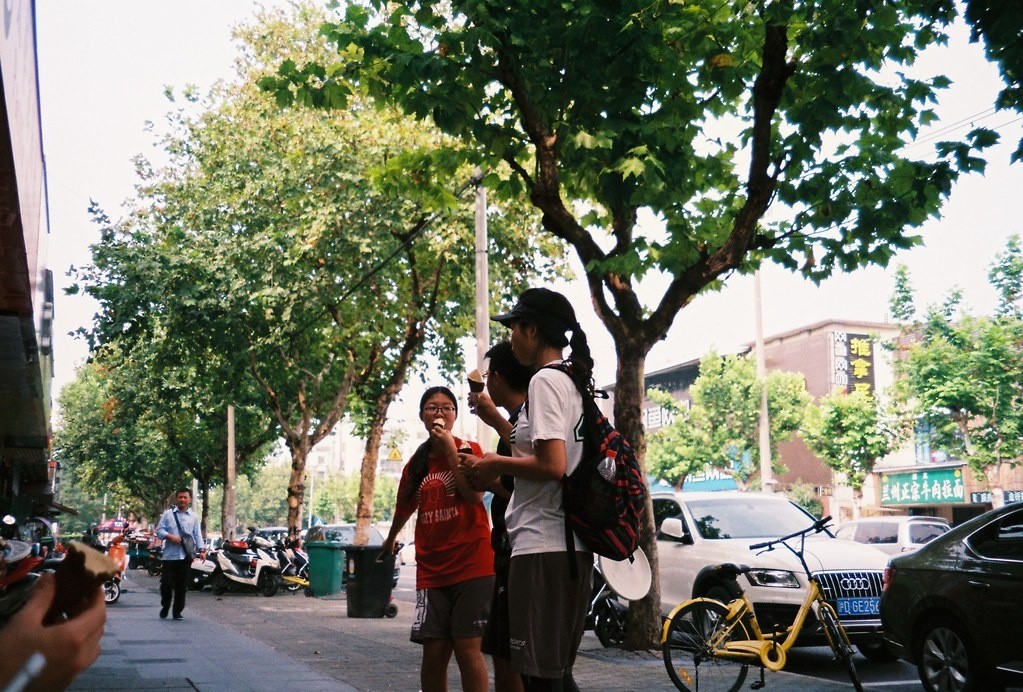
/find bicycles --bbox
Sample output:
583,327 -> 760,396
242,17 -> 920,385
658,513 -> 866,692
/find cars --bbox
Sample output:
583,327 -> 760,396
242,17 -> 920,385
301,521 -> 400,589
838,513 -> 953,557
248,526 -> 288,539
875,498 -> 1023,692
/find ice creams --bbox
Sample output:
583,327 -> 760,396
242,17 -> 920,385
42,541 -> 118,624
433,418 -> 445,430
467,368 -> 485,393
457,440 -> 473,455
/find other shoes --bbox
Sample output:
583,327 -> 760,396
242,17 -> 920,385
159,607 -> 169,618
173,614 -> 183,620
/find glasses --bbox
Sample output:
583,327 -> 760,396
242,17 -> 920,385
423,406 -> 456,415
481,373 -> 492,385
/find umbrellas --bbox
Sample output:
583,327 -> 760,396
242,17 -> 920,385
98,517 -> 137,531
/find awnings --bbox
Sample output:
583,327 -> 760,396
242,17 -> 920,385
52,502 -> 79,515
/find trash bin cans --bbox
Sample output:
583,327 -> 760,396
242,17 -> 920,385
303,542 -> 348,597
339,541 -> 404,618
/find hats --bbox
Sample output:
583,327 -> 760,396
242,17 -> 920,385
489,301 -> 570,329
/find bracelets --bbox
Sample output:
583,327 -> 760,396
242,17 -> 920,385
200,551 -> 205,553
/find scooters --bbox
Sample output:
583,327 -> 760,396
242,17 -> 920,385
0,513 -> 70,620
100,523 -> 133,604
126,521 -> 310,598
579,558 -> 631,649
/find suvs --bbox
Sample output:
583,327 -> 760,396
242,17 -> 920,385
650,487 -> 903,664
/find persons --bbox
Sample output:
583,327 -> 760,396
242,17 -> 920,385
83,528 -> 103,546
380,386 -> 497,692
457,342 -> 536,692
473,288 -> 595,692
156,488 -> 206,620
0,573 -> 107,692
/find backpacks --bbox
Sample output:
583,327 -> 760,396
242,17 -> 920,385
525,364 -> 645,564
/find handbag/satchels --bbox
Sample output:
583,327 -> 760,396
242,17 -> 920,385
182,533 -> 194,555
